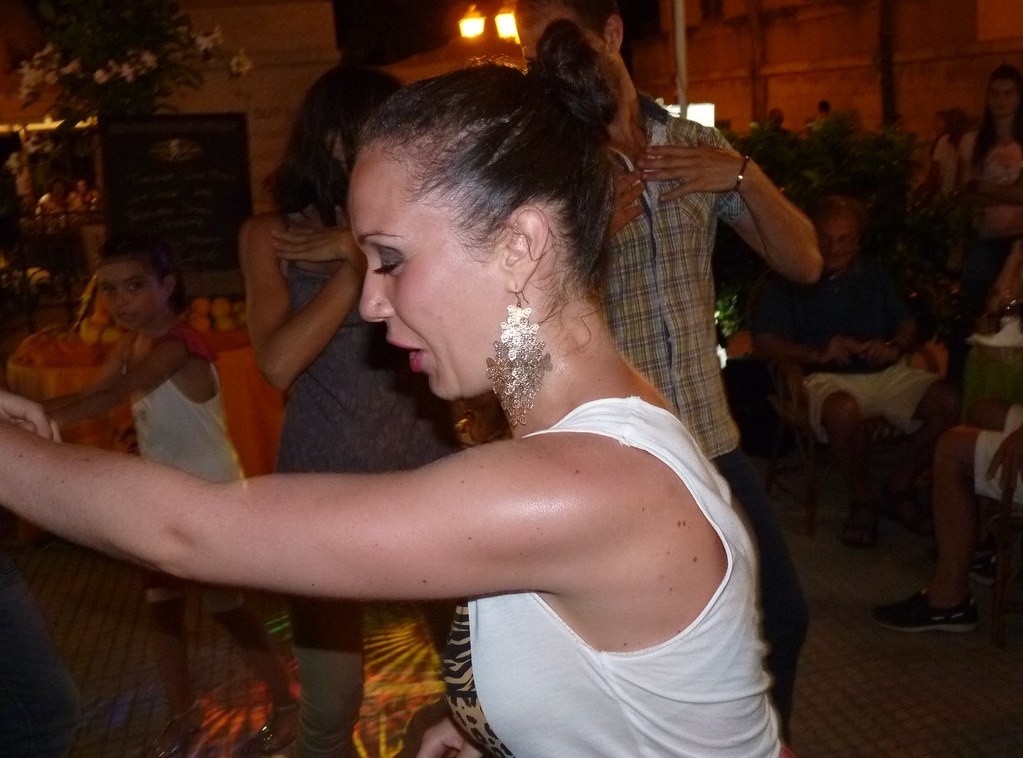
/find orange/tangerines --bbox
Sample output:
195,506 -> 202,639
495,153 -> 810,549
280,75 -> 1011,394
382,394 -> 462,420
188,296 -> 246,334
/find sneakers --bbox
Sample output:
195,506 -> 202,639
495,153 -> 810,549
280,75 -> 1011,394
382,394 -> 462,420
871,590 -> 979,633
926,544 -> 999,586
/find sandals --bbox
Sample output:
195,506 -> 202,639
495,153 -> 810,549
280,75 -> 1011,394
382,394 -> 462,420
882,490 -> 935,536
147,697 -> 205,758
841,504 -> 880,547
241,702 -> 302,754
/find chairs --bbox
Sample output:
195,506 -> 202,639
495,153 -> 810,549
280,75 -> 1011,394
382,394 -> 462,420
743,267 -> 921,539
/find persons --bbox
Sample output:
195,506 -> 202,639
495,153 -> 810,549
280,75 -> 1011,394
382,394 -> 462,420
238,62 -> 463,758
0,179 -> 304,758
745,64 -> 1023,631
515,0 -> 823,745
0,16 -> 789,758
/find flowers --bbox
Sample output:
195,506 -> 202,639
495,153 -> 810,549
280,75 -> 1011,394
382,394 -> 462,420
18,0 -> 254,97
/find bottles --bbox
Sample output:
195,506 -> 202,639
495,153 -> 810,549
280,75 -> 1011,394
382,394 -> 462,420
998,290 -> 1021,336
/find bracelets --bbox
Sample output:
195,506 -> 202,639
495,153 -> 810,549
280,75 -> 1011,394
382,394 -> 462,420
885,340 -> 900,350
734,156 -> 749,188
